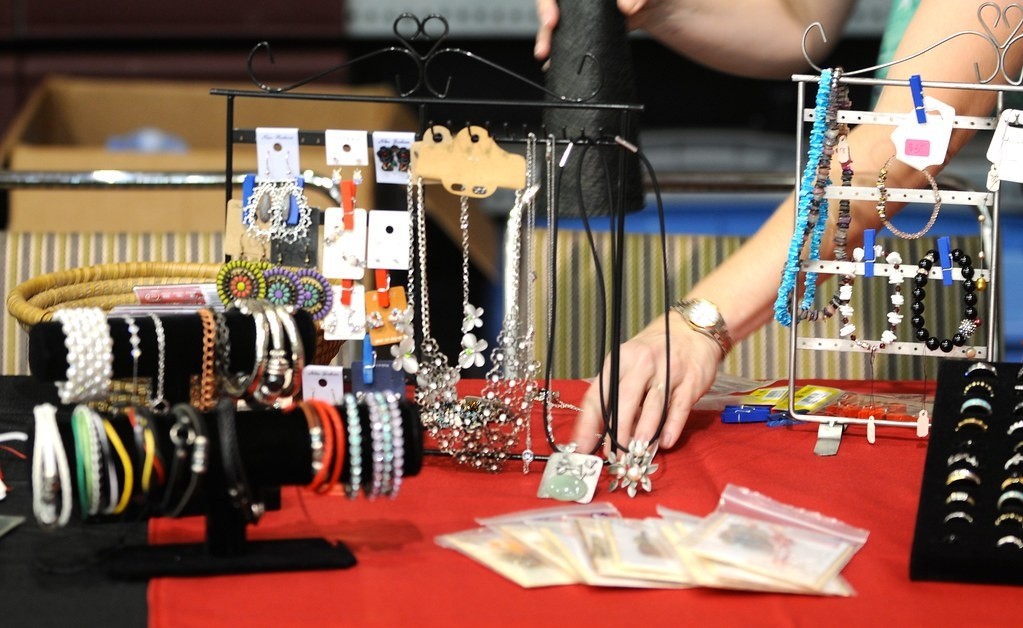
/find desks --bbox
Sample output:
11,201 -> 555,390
120,378 -> 1023,628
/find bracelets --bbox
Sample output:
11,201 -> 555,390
31,300 -> 403,528
838,245 -> 904,351
910,249 -> 982,353
877,156 -> 941,239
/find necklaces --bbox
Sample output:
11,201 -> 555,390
398,132 -> 670,503
773,67 -> 852,326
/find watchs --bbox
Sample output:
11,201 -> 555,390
668,299 -> 733,358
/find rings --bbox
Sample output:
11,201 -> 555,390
997,490 -> 1023,508
945,512 -> 973,523
1001,368 -> 1023,490
964,362 -> 998,377
962,380 -> 994,397
997,535 -> 1023,549
994,512 -> 1023,526
947,453 -> 979,468
946,492 -> 974,505
946,469 -> 981,486
959,439 -> 976,447
960,398 -> 992,415
955,418 -> 988,432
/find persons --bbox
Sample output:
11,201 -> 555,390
535,0 -> 1023,458
